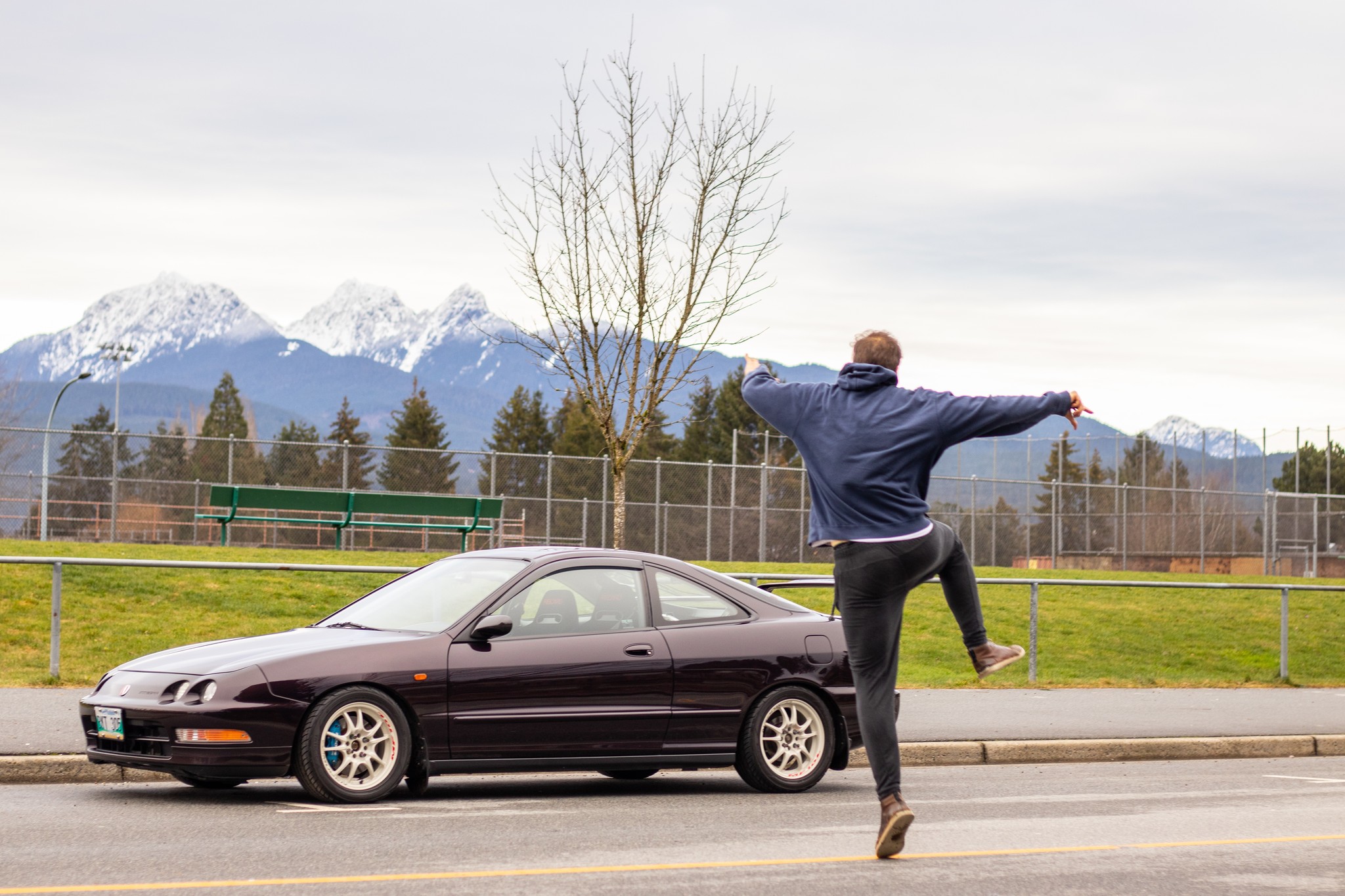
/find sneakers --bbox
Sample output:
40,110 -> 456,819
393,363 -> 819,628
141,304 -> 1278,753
875,790 -> 915,858
967,639 -> 1026,680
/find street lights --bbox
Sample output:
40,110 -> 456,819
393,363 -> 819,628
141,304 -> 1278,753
97,340 -> 136,542
40,373 -> 93,540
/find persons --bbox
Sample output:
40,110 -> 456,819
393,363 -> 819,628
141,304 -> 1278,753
740,328 -> 1098,861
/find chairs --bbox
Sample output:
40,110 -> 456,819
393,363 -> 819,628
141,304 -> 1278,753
568,584 -> 640,634
512,589 -> 580,637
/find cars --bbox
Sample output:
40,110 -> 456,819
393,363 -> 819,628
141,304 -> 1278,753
79,546 -> 902,806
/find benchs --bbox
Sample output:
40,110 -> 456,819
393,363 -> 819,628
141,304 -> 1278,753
194,485 -> 503,552
660,602 -> 727,623
